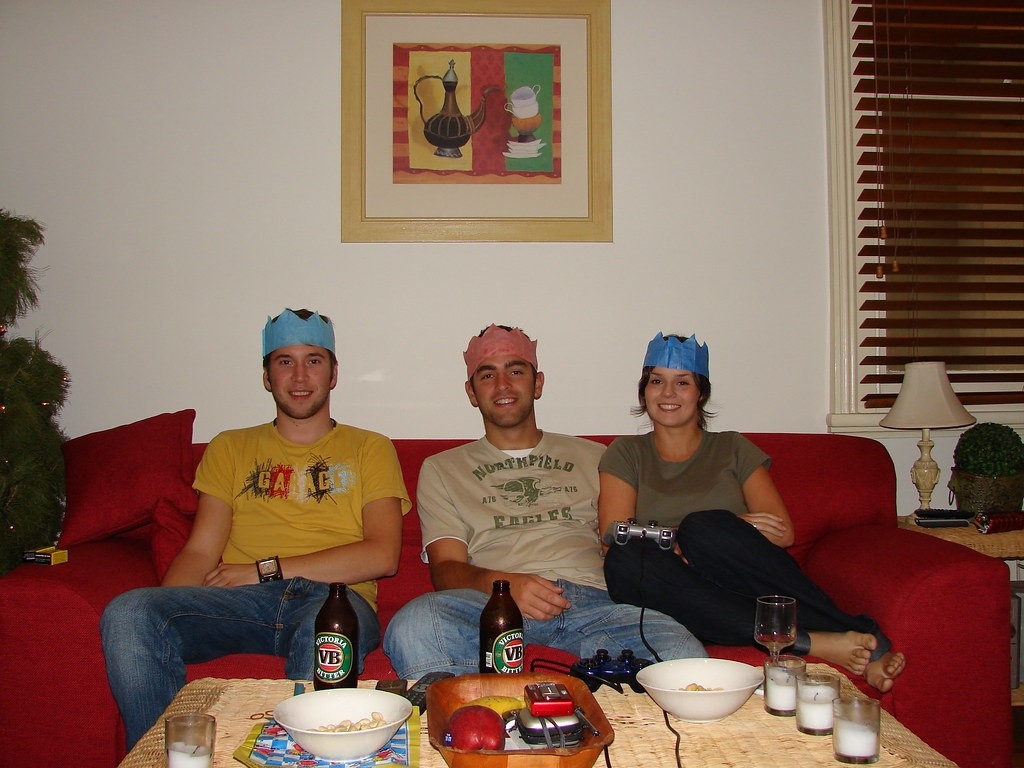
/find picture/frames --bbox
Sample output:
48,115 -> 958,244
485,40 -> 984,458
340,0 -> 614,244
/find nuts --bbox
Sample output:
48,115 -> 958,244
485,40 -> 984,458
685,683 -> 724,691
307,712 -> 389,734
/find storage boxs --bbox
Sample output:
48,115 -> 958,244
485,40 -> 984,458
23,545 -> 56,560
34,549 -> 69,566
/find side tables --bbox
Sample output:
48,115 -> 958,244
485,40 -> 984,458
898,516 -> 1024,753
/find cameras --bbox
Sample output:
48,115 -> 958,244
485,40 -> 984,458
524,682 -> 574,717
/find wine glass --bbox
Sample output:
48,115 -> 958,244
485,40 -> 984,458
754,596 -> 797,660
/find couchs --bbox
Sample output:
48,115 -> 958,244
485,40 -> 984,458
0,432 -> 1015,768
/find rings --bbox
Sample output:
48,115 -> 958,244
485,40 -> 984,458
753,522 -> 757,528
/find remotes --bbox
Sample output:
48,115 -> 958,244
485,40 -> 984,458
404,672 -> 455,716
915,508 -> 974,519
915,518 -> 971,528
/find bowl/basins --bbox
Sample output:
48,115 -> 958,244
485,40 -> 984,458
272,688 -> 413,761
635,657 -> 766,722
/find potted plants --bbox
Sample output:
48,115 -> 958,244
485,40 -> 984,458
947,422 -> 1024,524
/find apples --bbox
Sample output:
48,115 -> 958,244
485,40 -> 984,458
446,704 -> 497,751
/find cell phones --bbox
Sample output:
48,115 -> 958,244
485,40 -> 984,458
376,679 -> 408,698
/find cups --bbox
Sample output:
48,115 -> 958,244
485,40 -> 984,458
763,654 -> 807,716
794,671 -> 840,735
165,712 -> 217,768
831,698 -> 882,765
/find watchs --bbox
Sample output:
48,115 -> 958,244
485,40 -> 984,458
256,555 -> 284,583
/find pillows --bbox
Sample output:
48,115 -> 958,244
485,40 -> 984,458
149,494 -> 194,579
54,408 -> 199,549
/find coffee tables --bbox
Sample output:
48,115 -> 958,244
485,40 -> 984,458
117,662 -> 959,768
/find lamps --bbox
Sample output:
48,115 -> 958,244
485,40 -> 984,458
878,361 -> 977,526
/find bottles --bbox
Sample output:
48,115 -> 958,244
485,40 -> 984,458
313,582 -> 358,692
479,579 -> 523,676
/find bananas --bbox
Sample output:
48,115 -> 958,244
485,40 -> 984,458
469,695 -> 527,716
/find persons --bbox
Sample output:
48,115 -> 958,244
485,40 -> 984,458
98,308 -> 412,754
383,325 -> 708,679
598,335 -> 906,693
